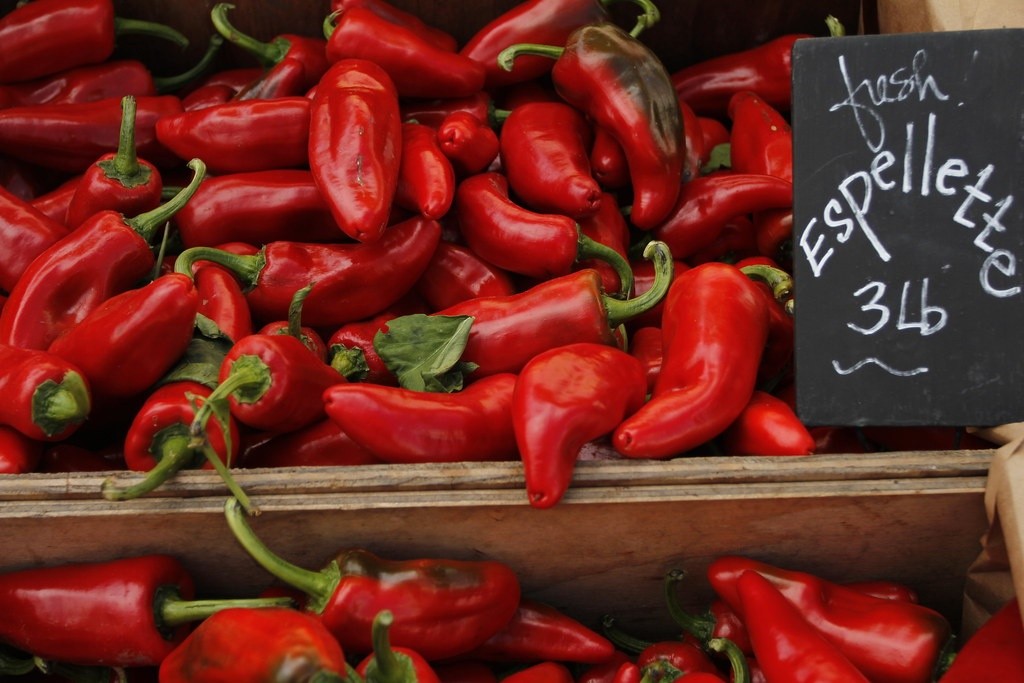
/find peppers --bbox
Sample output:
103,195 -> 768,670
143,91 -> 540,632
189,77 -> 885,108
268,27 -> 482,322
0,0 -> 1024,683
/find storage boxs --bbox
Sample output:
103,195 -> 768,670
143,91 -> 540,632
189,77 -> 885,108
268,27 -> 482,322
0,0 -> 1024,643
